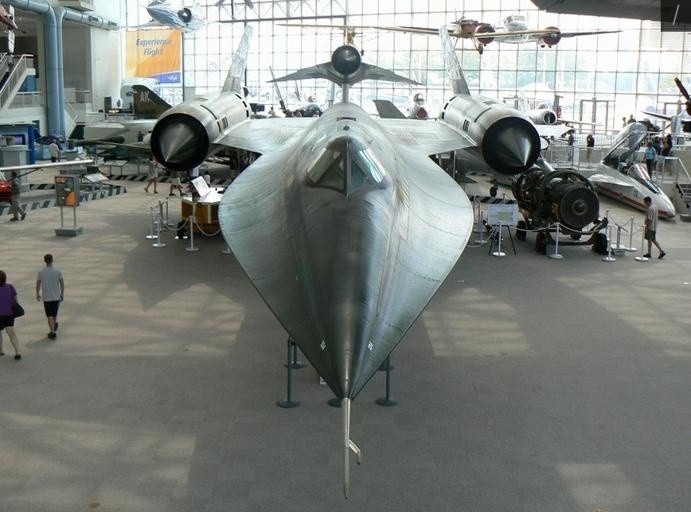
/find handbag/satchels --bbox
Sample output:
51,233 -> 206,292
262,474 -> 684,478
13,302 -> 24,317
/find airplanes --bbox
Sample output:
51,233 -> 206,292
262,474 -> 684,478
74,8 -> 622,510
588,118 -> 678,222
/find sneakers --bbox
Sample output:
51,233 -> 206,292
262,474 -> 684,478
0,353 -> 5,355
11,213 -> 26,221
15,355 -> 21,360
48,323 -> 58,339
658,253 -> 665,259
643,254 -> 651,257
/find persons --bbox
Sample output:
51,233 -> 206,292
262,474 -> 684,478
623,117 -> 628,128
10,171 -> 27,221
144,154 -> 160,193
267,106 -> 276,118
36,253 -> 65,338
586,134 -> 594,169
644,134 -> 673,178
169,169 -> 187,196
49,139 -> 60,162
628,114 -> 637,125
643,196 -> 666,259
0,270 -> 22,359
568,131 -> 575,161
